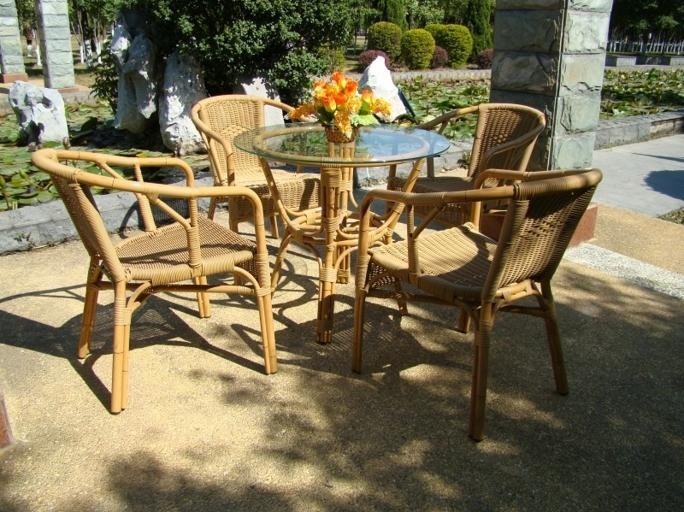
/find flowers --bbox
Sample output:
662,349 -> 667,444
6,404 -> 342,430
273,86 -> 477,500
284,71 -> 392,144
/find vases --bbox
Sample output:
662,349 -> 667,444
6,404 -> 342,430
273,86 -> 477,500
325,121 -> 357,143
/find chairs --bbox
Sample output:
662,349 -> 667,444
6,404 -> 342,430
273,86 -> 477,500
192,94 -> 342,302
31,147 -> 277,414
351,168 -> 602,441
388,103 -> 547,331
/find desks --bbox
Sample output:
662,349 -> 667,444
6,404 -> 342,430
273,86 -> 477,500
234,122 -> 452,346
